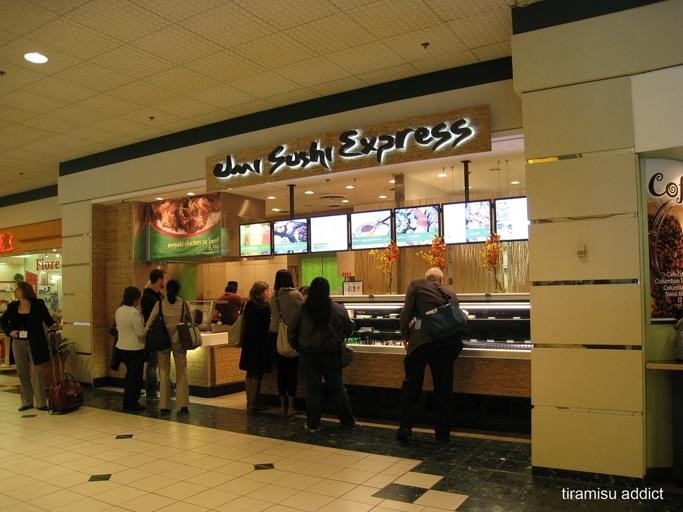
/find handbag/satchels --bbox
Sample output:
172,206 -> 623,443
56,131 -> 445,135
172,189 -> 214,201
177,322 -> 202,350
425,302 -> 464,340
276,319 -> 297,358
342,343 -> 353,368
146,316 -> 172,351
228,315 -> 243,345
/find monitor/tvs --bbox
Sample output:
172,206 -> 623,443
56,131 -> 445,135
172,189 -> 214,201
442,199 -> 492,245
238,221 -> 272,257
310,213 -> 349,253
350,208 -> 393,251
494,196 -> 530,242
273,217 -> 309,256
394,204 -> 441,248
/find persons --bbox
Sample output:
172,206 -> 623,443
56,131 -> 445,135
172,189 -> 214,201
0,281 -> 62,411
396,267 -> 463,442
111,269 -> 357,433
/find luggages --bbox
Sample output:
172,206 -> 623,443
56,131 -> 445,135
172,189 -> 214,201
47,330 -> 84,415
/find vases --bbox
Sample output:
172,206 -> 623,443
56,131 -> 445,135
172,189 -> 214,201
491,266 -> 504,293
383,267 -> 394,296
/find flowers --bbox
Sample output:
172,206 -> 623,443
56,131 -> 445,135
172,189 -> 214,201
474,230 -> 508,293
367,239 -> 402,296
412,233 -> 450,287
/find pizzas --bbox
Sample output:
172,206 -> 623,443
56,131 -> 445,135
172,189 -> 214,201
148,193 -> 222,234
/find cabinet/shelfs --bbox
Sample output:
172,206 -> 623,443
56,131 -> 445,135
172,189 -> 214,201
167,298 -> 247,398
0,280 -> 17,316
323,291 -> 534,403
